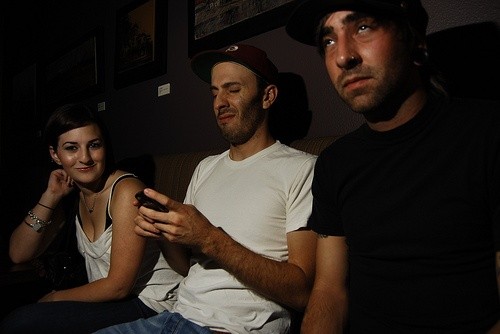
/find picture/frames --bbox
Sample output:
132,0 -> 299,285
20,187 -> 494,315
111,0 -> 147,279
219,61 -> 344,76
112,0 -> 168,90
187,0 -> 304,61
43,25 -> 105,113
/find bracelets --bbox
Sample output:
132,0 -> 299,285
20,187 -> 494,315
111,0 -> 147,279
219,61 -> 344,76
24,202 -> 54,232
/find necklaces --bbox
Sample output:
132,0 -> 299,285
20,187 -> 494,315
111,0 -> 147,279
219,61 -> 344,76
82,194 -> 96,216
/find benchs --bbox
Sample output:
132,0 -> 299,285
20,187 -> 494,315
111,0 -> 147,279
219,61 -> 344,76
144,134 -> 344,205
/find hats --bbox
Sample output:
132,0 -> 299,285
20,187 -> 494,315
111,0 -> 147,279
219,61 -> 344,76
283,0 -> 429,46
191,42 -> 278,83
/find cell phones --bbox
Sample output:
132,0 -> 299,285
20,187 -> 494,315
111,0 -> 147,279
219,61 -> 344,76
134,190 -> 169,212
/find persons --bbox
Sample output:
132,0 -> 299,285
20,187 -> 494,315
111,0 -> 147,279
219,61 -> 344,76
285,0 -> 500,334
0,104 -> 183,334
94,44 -> 318,334
